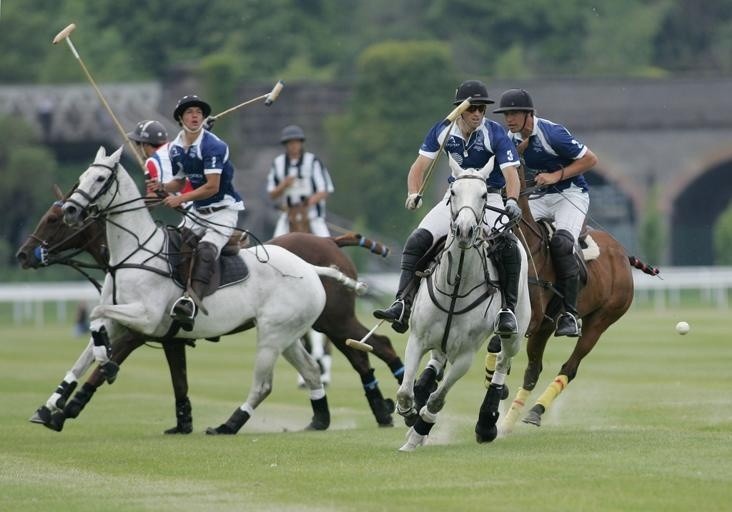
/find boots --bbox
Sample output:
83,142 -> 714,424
489,234 -> 521,332
487,335 -> 502,354
174,241 -> 218,332
551,231 -> 580,337
373,228 -> 434,334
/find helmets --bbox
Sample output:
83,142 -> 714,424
280,124 -> 306,143
173,94 -> 211,122
453,79 -> 495,105
128,119 -> 168,146
492,88 -> 535,114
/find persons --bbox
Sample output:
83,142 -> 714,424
144,92 -> 246,332
486,87 -> 599,354
266,124 -> 337,241
126,116 -> 196,237
371,80 -> 523,340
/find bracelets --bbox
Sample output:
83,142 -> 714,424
506,197 -> 518,204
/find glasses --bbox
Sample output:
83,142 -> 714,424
465,105 -> 486,113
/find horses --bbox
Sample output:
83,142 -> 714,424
394,147 -> 534,453
27,143 -> 378,435
14,184 -> 409,437
278,193 -> 335,388
479,135 -> 661,440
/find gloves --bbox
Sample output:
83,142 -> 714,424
506,197 -> 523,222
203,116 -> 216,130
405,192 -> 422,211
